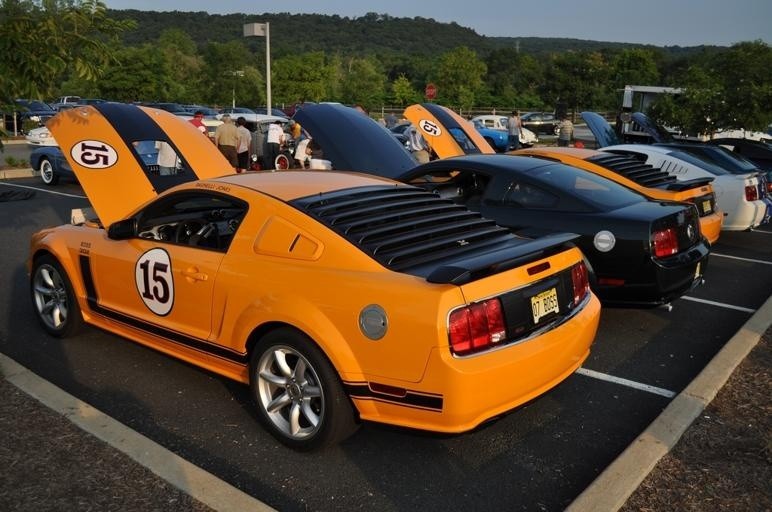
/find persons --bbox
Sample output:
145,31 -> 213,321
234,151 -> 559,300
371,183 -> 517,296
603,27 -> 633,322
214,113 -> 241,174
465,115 -> 477,150
249,124 -> 267,171
554,114 -> 575,147
286,119 -> 302,149
505,110 -> 525,152
154,140 -> 182,176
264,120 -> 285,170
235,116 -> 252,173
379,112 -> 436,165
188,110 -> 210,138
295,127 -> 316,169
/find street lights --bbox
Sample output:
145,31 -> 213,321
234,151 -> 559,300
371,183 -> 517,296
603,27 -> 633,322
223,21 -> 272,116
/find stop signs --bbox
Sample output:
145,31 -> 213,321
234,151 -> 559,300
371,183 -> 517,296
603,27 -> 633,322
425,84 -> 436,99
